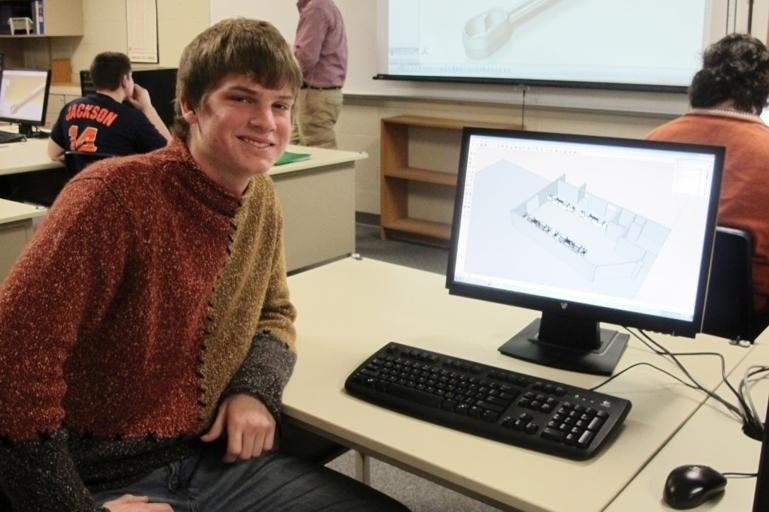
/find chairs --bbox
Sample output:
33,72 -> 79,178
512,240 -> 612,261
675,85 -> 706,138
699,223 -> 769,342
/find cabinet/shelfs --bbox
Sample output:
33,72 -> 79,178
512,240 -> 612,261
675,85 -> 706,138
380,114 -> 527,243
0,0 -> 84,41
44,82 -> 82,131
80,68 -> 178,126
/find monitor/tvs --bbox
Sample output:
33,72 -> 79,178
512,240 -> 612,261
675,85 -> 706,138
444,126 -> 726,376
0,68 -> 52,138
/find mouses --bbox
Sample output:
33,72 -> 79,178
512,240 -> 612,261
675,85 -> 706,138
663,464 -> 727,509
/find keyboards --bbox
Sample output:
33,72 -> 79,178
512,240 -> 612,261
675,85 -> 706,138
344,341 -> 631,462
0,130 -> 26,144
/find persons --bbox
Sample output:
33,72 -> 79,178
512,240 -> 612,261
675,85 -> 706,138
0,16 -> 413,512
44,52 -> 177,181
289,0 -> 348,151
638,34 -> 769,323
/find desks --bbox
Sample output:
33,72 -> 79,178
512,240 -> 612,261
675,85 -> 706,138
601,344 -> 769,511
280,253 -> 754,512
